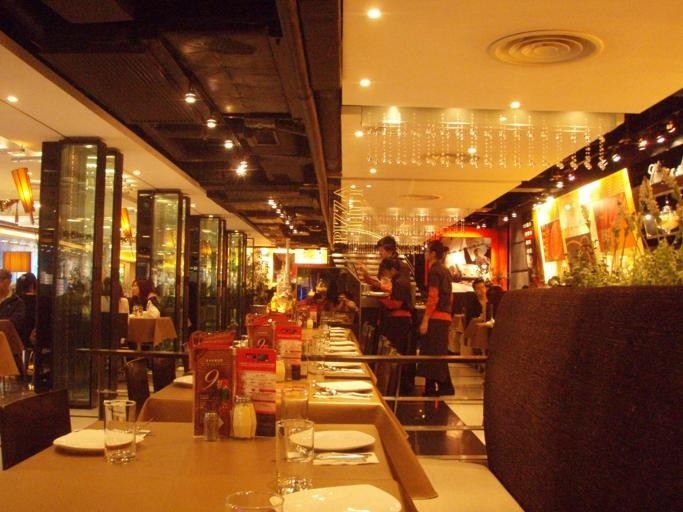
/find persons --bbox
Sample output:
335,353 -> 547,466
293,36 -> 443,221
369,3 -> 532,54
333,292 -> 357,312
365,236 -> 488,396
0,269 -> 162,369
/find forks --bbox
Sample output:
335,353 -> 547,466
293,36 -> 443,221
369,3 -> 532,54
297,449 -> 380,461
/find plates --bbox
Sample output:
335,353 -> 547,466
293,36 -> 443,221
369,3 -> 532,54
293,431 -> 378,451
171,373 -> 195,390
285,481 -> 401,511
49,428 -> 130,456
323,323 -> 371,395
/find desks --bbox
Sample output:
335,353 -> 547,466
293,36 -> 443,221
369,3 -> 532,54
448,314 -> 493,372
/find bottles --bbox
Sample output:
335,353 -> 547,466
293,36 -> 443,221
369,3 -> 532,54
641,157 -> 683,238
203,411 -> 217,441
234,394 -> 258,439
129,306 -> 146,317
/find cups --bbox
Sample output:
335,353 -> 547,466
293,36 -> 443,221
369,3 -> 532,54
231,334 -> 248,352
224,491 -> 284,511
274,386 -> 314,491
103,398 -> 136,462
288,326 -> 325,396
